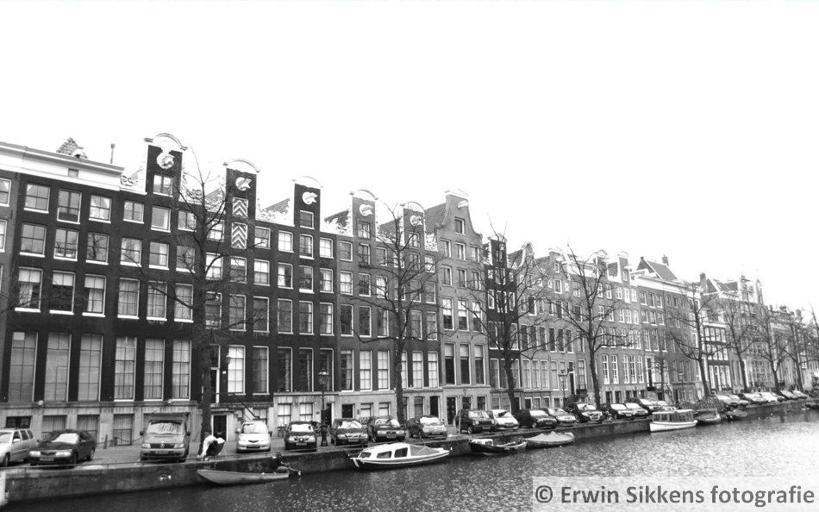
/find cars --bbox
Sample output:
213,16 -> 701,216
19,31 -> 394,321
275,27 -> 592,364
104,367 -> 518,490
26,429 -> 97,469
284,421 -> 317,452
714,389 -> 808,411
406,415 -> 446,440
367,415 -> 406,442
609,397 -> 677,420
455,402 -> 606,435
235,420 -> 272,453
329,418 -> 369,447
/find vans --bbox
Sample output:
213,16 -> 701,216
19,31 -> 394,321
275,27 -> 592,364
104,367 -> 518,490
139,420 -> 190,463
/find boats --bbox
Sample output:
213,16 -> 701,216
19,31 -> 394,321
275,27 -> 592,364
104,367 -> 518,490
349,442 -> 450,471
196,469 -> 289,485
805,398 -> 819,410
468,431 -> 575,458
649,408 -> 747,432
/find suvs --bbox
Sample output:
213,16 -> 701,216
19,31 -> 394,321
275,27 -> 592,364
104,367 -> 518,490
0,428 -> 36,467
